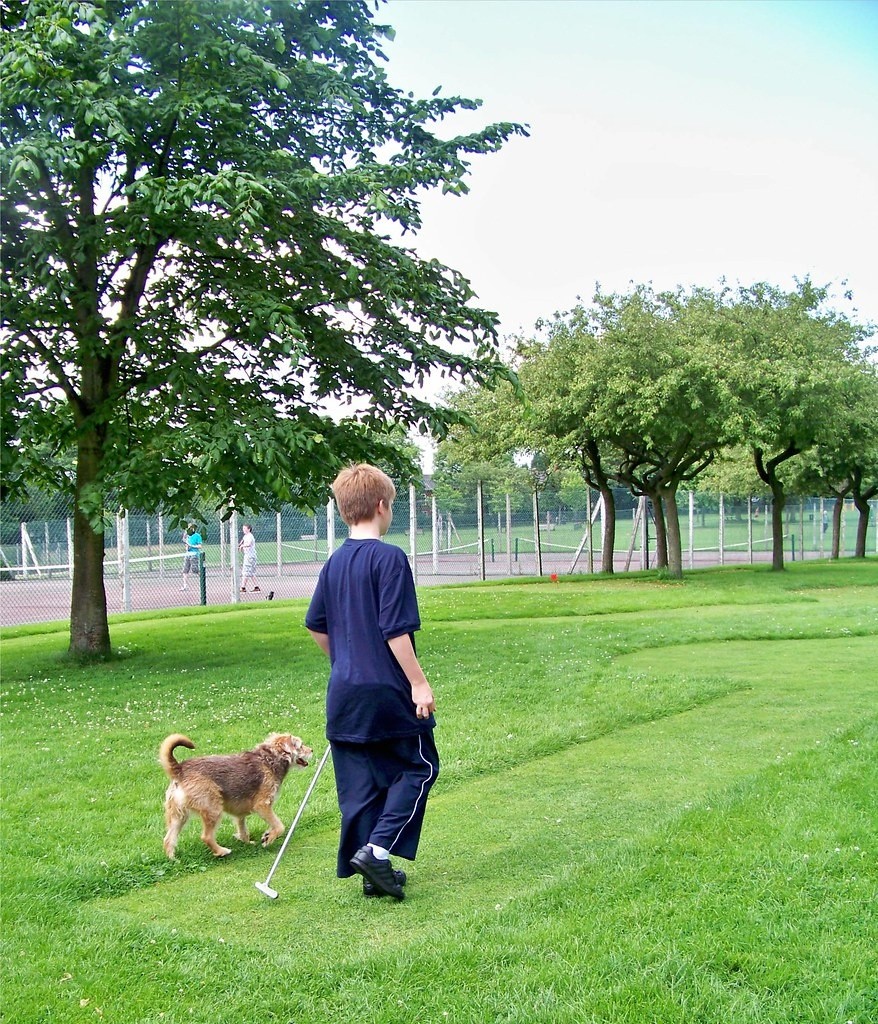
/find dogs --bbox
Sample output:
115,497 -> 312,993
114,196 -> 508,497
159,733 -> 314,862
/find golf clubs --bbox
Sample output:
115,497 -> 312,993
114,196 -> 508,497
252,743 -> 331,901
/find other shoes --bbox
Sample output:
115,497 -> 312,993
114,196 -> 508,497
179,586 -> 188,591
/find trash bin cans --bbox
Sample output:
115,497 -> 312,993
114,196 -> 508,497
809,514 -> 813,520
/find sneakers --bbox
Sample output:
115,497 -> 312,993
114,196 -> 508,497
249,587 -> 260,593
240,587 -> 246,594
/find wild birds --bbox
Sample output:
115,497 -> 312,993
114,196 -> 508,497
265,591 -> 274,600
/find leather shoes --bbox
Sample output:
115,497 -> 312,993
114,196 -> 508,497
350,846 -> 406,900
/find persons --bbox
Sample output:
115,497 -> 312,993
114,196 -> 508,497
305,463 -> 439,902
870,504 -> 875,527
238,523 -> 261,594
755,507 -> 759,518
178,523 -> 202,590
823,511 -> 828,535
